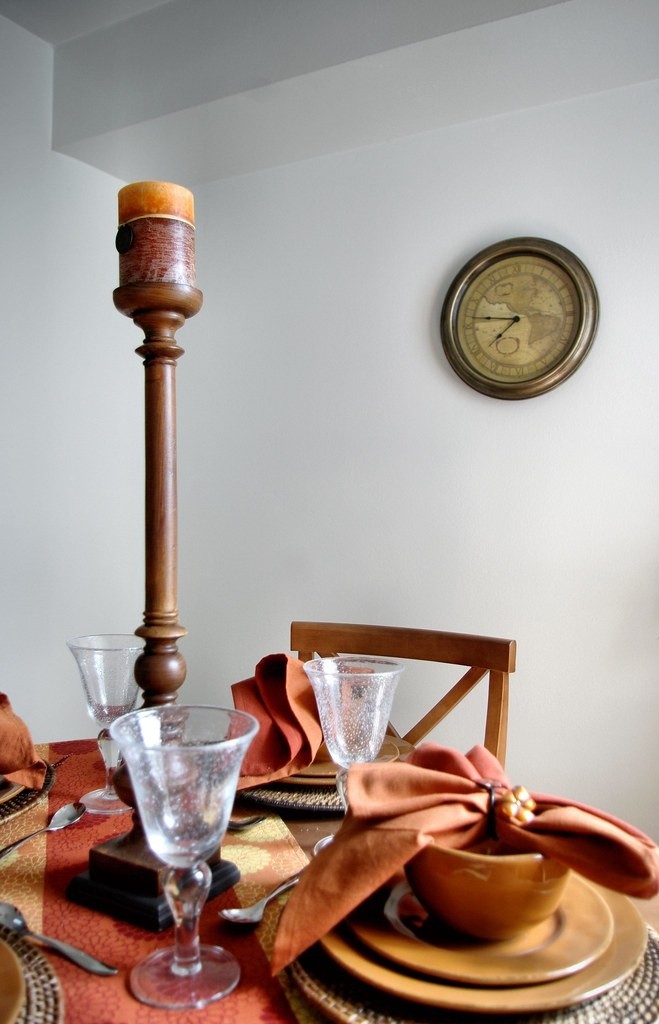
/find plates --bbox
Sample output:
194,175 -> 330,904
0,781 -> 25,803
346,865 -> 614,984
291,739 -> 399,776
316,866 -> 647,1014
274,735 -> 414,785
0,938 -> 25,1024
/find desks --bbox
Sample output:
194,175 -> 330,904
0,740 -> 659,1024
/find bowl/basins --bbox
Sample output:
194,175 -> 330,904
404,832 -> 569,941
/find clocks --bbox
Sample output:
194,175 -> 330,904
438,235 -> 599,399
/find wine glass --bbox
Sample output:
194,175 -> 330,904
304,655 -> 404,855
108,704 -> 261,1007
66,634 -> 146,814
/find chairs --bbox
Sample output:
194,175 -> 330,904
289,619 -> 515,770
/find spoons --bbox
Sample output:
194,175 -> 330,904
227,816 -> 266,830
217,876 -> 301,922
0,803 -> 86,859
0,902 -> 118,975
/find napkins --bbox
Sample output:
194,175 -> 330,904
0,692 -> 48,789
231,652 -> 373,791
257,744 -> 659,975
